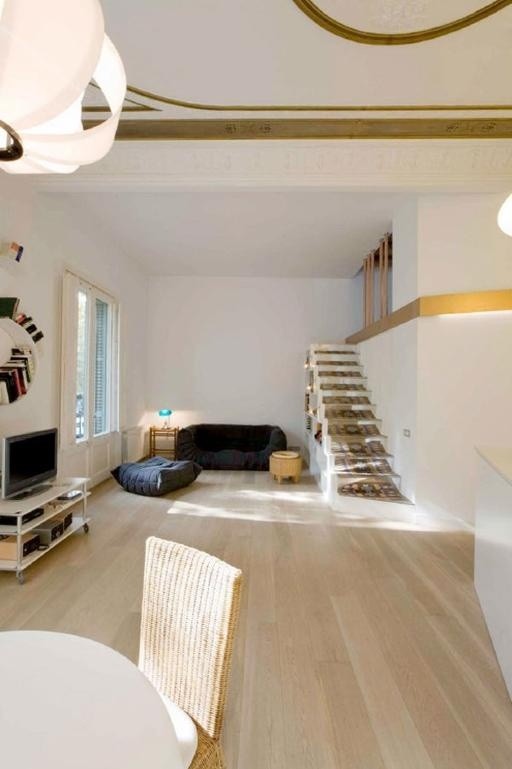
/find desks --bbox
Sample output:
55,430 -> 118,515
0,630 -> 186,769
473,447 -> 512,703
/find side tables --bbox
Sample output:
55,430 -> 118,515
150,426 -> 179,460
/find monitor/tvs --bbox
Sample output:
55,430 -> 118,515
1,428 -> 57,501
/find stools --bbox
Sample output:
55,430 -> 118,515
269,450 -> 302,484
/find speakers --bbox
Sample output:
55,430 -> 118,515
0,510 -> 73,560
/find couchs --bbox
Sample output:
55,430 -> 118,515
176,425 -> 287,470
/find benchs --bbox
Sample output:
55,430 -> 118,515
111,455 -> 203,496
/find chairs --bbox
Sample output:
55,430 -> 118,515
138,536 -> 243,769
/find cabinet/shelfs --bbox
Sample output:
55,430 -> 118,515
0,242 -> 43,408
0,477 -> 91,585
303,344 -> 415,522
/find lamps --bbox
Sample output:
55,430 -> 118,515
497,193 -> 512,237
0,0 -> 127,175
159,409 -> 172,429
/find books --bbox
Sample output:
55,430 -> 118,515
1,296 -> 44,404
